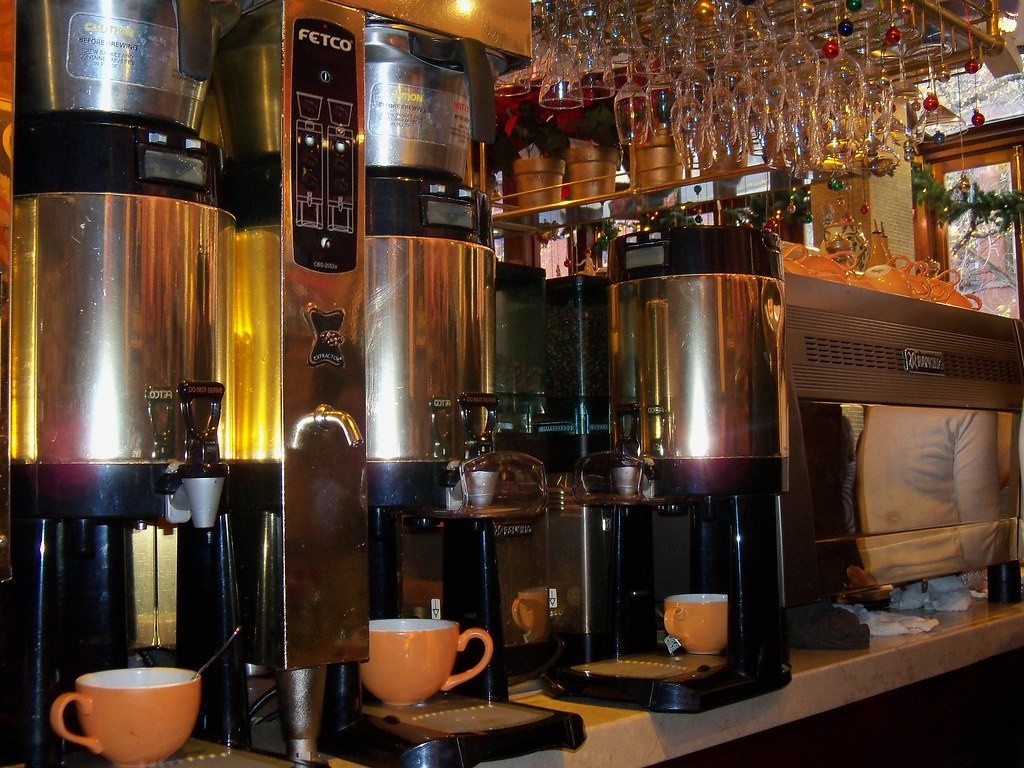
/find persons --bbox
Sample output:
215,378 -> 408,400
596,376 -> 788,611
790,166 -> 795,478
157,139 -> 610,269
856,404 -> 1009,584
800,400 -> 867,590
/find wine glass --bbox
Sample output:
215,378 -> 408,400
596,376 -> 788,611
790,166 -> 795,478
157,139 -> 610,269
494,0 -> 968,180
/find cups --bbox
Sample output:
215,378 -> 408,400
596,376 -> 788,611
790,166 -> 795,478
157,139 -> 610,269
51,667 -> 201,768
664,594 -> 728,654
361,618 -> 493,705
782,243 -> 983,311
512,589 -> 548,639
